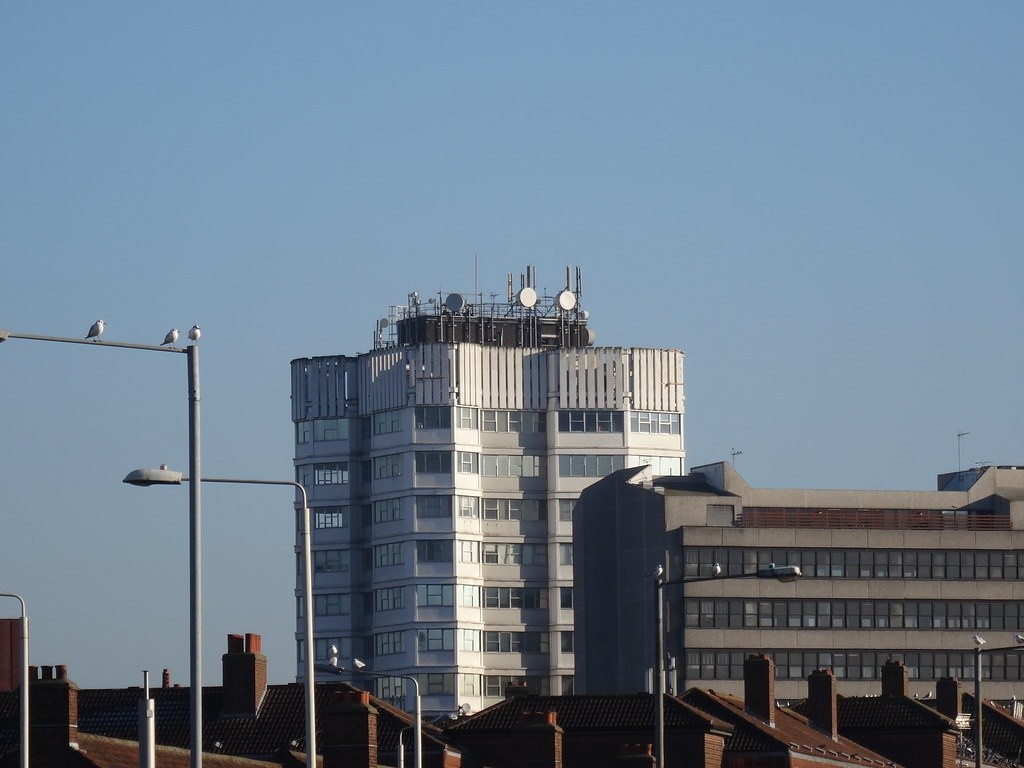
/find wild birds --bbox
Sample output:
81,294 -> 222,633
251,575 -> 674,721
642,563 -> 663,579
913,693 -> 919,699
971,636 -> 986,647
920,691 -> 932,700
85,319 -> 107,342
1014,634 -> 1024,646
187,324 -> 202,346
328,644 -> 338,661
160,328 -> 181,350
353,658 -> 366,671
711,563 -> 721,577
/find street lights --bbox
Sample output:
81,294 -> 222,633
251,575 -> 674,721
302,663 -> 422,768
122,469 -> 316,768
398,712 -> 458,768
655,565 -> 804,768
